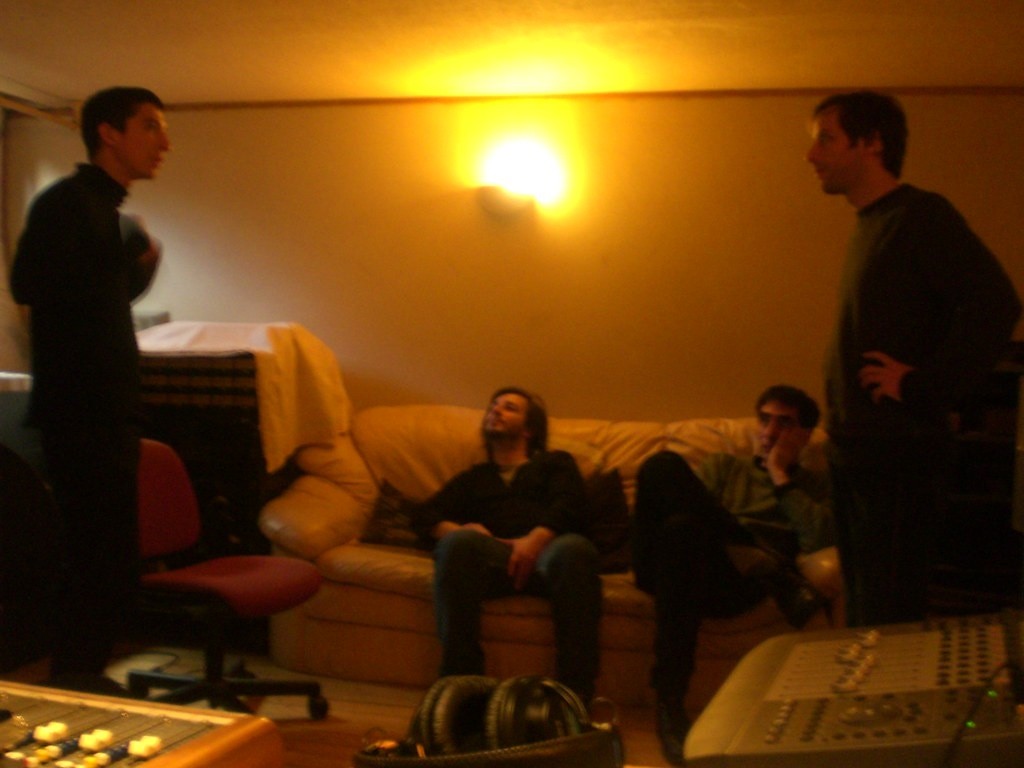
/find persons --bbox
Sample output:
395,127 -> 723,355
629,384 -> 836,767
806,90 -> 1024,634
10,86 -> 171,697
407,386 -> 604,717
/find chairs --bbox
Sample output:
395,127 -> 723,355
127,438 -> 333,718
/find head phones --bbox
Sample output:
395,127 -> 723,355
352,674 -> 625,768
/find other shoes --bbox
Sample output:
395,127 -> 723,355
656,699 -> 693,766
47,665 -> 123,699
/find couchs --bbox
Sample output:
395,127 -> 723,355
258,406 -> 840,717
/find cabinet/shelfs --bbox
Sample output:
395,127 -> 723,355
1,374 -> 55,665
137,355 -> 299,651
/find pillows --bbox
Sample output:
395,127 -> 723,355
357,480 -> 429,549
587,469 -> 635,575
